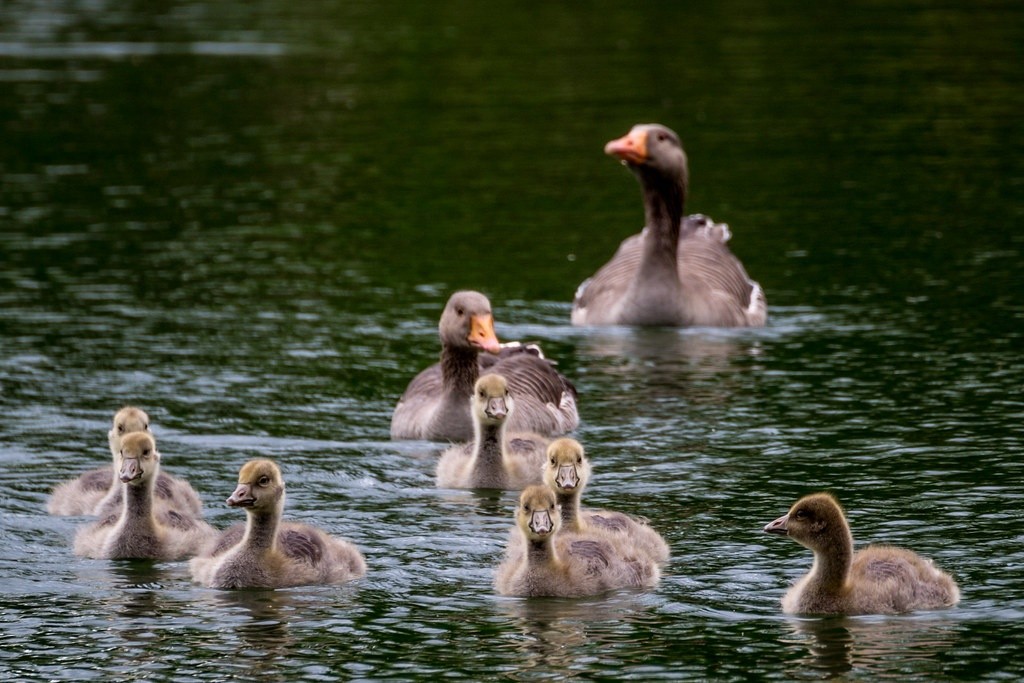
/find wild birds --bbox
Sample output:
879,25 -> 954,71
566,118 -> 772,335
34,401 -> 373,599
767,490 -> 970,629
387,283 -> 677,606
